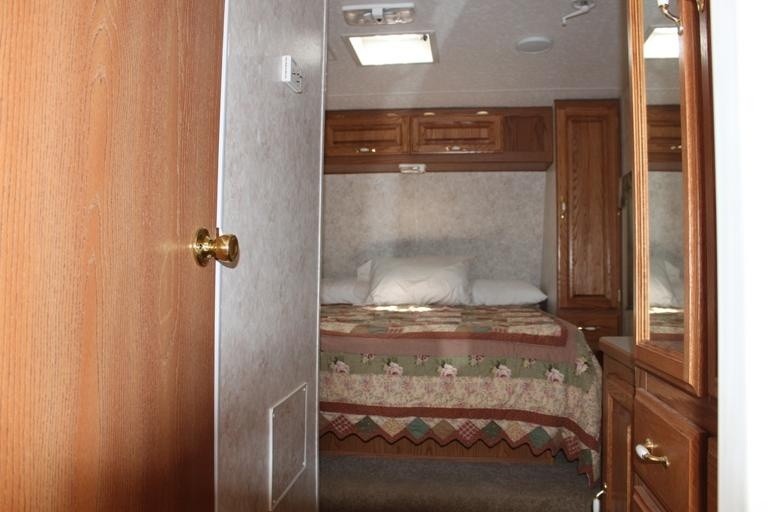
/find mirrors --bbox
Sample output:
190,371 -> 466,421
626,0 -> 704,399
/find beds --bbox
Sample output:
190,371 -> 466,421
319,304 -> 575,465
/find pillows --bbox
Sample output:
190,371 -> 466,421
319,256 -> 548,306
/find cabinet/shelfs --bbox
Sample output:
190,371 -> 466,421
596,337 -> 717,512
324,107 -> 553,175
541,98 -> 622,362
